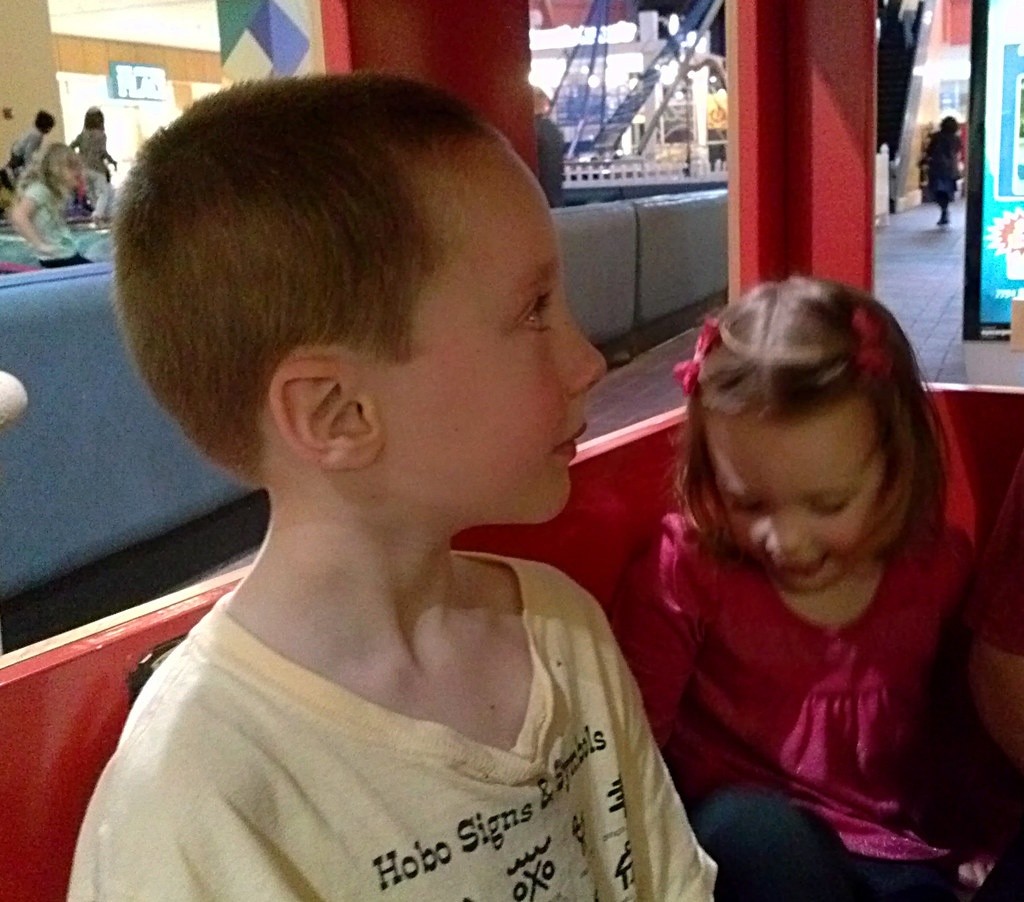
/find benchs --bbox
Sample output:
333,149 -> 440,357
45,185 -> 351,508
0,385 -> 1024,902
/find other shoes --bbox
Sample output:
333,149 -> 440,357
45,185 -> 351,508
937,218 -> 947,224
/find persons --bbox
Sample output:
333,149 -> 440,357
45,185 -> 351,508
920,116 -> 962,225
66,68 -> 719,902
534,89 -> 564,209
0,107 -> 117,268
608,273 -> 1024,902
